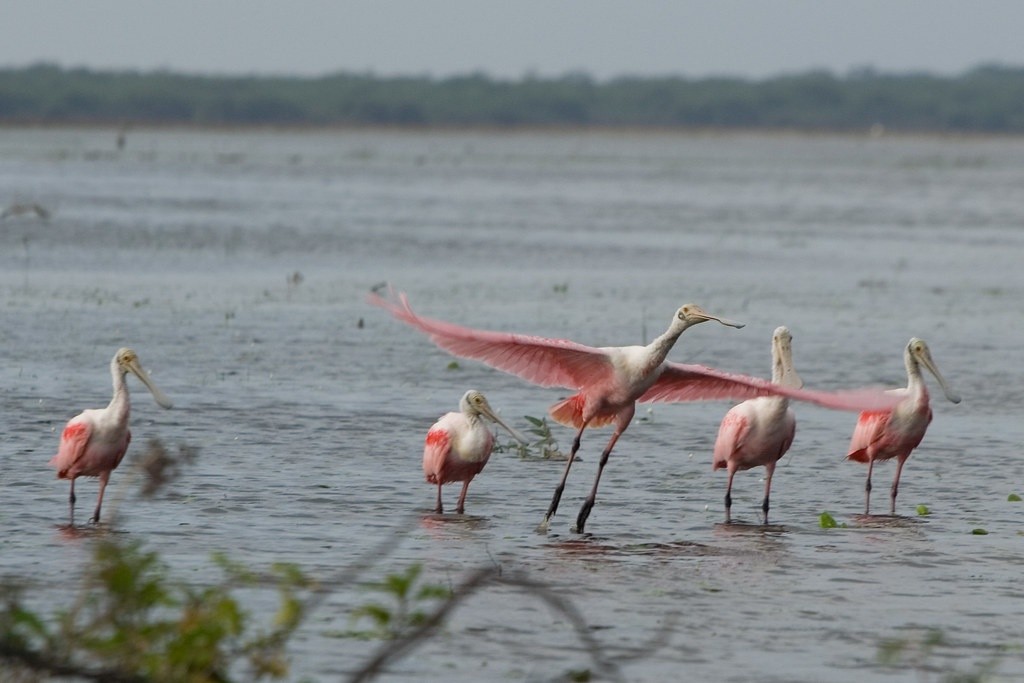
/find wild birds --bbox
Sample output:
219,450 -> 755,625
847,335 -> 962,524
712,323 -> 804,525
424,388 -> 530,514
50,346 -> 173,528
366,293 -> 906,535
